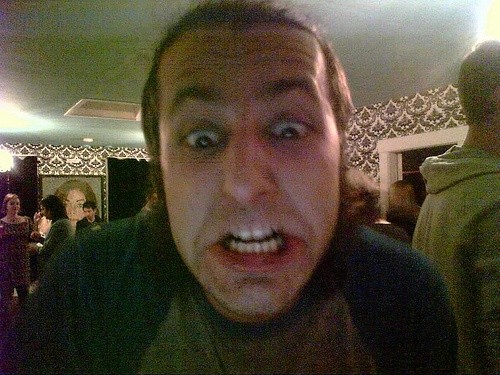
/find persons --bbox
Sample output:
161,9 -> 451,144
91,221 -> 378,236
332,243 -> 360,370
54,178 -> 96,234
341,167 -> 419,246
76,201 -> 105,234
141,189 -> 158,210
0,192 -> 33,301
408,41 -> 500,375
13,0 -> 459,375
27,195 -> 71,265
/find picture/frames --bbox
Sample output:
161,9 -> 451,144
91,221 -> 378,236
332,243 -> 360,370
36,173 -> 107,222
